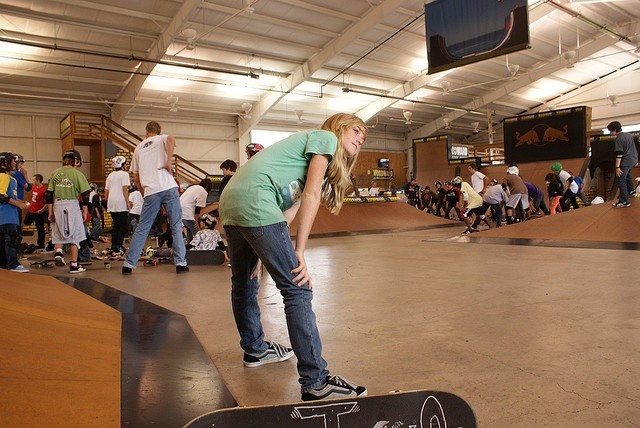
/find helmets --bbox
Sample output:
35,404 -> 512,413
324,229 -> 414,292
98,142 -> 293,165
444,180 -> 452,187
550,162 -> 562,173
14,153 -> 24,170
0,152 -> 16,172
111,155 -> 126,169
435,180 -> 442,186
451,176 -> 463,185
89,182 -> 97,190
506,165 -> 519,176
200,213 -> 218,226
634,176 -> 639,182
246,142 -> 263,153
63,149 -> 82,168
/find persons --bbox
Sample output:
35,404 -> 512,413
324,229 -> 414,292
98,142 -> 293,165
550,162 -> 579,211
121,120 -> 189,274
23,173 -> 49,251
501,166 -> 531,223
146,213 -> 231,262
568,171 -> 589,208
155,221 -> 173,248
544,172 -> 563,215
403,192 -> 410,204
245,142 -> 264,161
405,178 -> 416,206
472,182 -> 509,229
433,180 -> 447,216
90,188 -> 104,241
12,153 -> 33,261
427,191 -> 438,213
414,180 -> 421,210
606,121 -> 639,208
452,176 -> 493,235
178,178 -> 213,244
123,181 -> 144,240
213,159 -> 238,267
507,163 -> 523,179
0,151 -> 30,274
630,176 -> 640,198
104,155 -> 132,253
369,181 -> 379,197
389,176 -> 397,195
88,183 -> 98,204
443,182 -> 465,221
420,186 -> 431,213
523,182 -> 550,215
466,164 -> 489,232
217,113 -> 369,403
76,200 -> 94,266
45,149 -> 92,273
489,178 -> 502,221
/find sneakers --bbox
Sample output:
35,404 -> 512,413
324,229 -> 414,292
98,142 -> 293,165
10,265 -> 30,273
69,261 -> 86,274
628,190 -> 637,197
301,374 -> 368,401
612,201 -> 631,208
122,266 -> 132,274
175,266 -> 190,274
241,338 -> 294,367
462,230 -> 472,235
54,251 -> 66,266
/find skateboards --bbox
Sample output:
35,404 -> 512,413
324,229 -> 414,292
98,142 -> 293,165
170,250 -> 225,265
183,389 -> 478,428
28,243 -> 171,270
